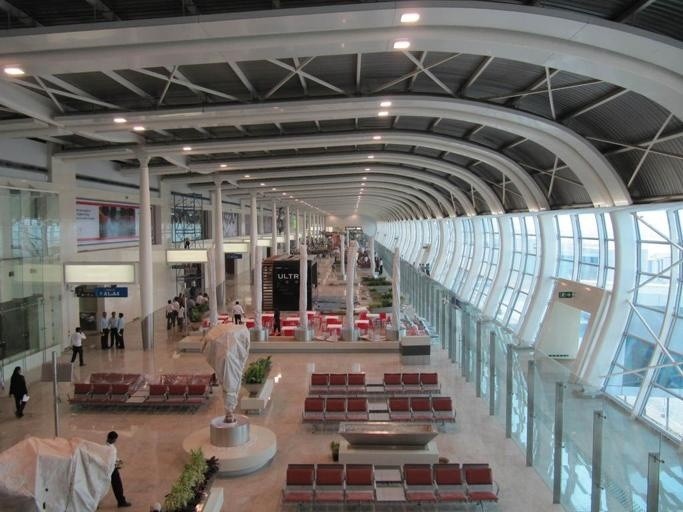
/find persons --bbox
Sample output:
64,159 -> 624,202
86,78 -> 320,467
166,324 -> 189,359
412,261 -> 431,277
268,304 -> 281,335
106,312 -> 118,348
98,311 -> 110,350
71,364 -> 87,382
164,291 -> 207,328
115,312 -> 124,349
7,366 -> 28,419
103,429 -> 132,507
98,346 -> 123,369
69,327 -> 87,366
232,300 -> 246,324
356,249 -> 382,275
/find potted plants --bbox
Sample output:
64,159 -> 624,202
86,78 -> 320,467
161,447 -> 224,512
239,355 -> 274,394
189,302 -> 208,331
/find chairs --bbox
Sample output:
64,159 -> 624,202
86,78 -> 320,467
281,372 -> 500,511
69,373 -> 216,412
201,306 -> 388,341
